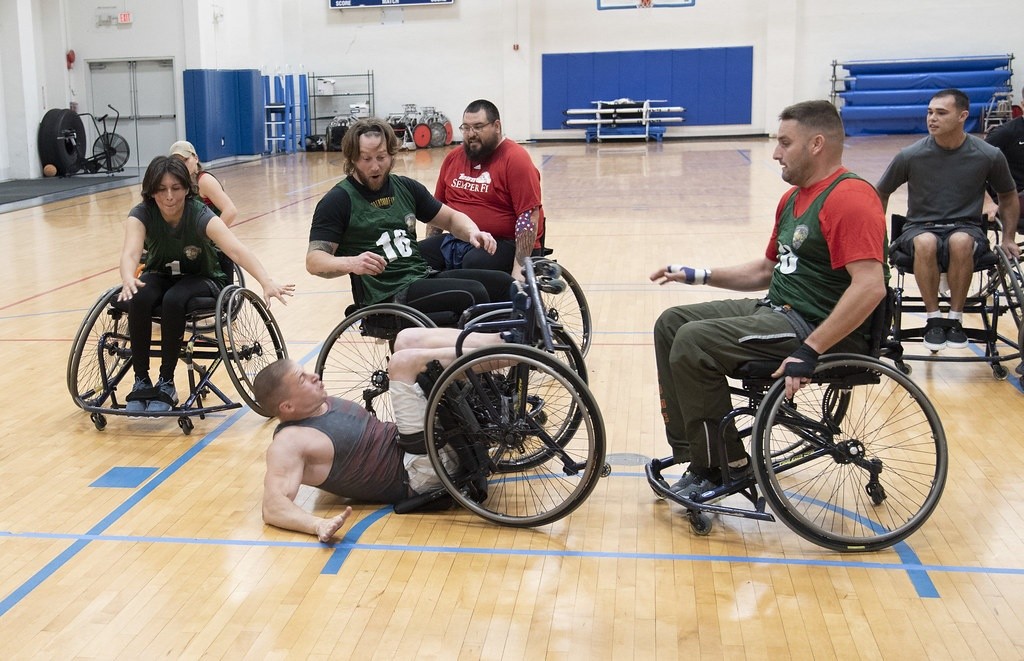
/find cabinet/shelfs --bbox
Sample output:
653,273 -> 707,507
307,70 -> 374,137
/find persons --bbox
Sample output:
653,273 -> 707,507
253,281 -> 531,543
118,156 -> 295,418
416,100 -> 546,285
874,89 -> 1022,349
306,116 -> 524,333
650,100 -> 890,504
984,116 -> 1024,235
170,141 -> 238,228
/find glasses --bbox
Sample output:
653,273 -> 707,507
460,122 -> 496,135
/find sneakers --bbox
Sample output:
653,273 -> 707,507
946,320 -> 968,348
500,292 -> 532,349
125,375 -> 153,420
145,378 -> 179,419
676,453 -> 753,500
509,280 -> 524,299
667,465 -> 704,492
923,315 -> 947,350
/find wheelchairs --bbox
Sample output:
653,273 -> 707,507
644,284 -> 950,554
313,216 -> 612,527
68,242 -> 290,435
888,211 -> 1024,390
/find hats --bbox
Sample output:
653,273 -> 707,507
168,140 -> 197,160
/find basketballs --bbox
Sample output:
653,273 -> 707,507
43,164 -> 57,177
